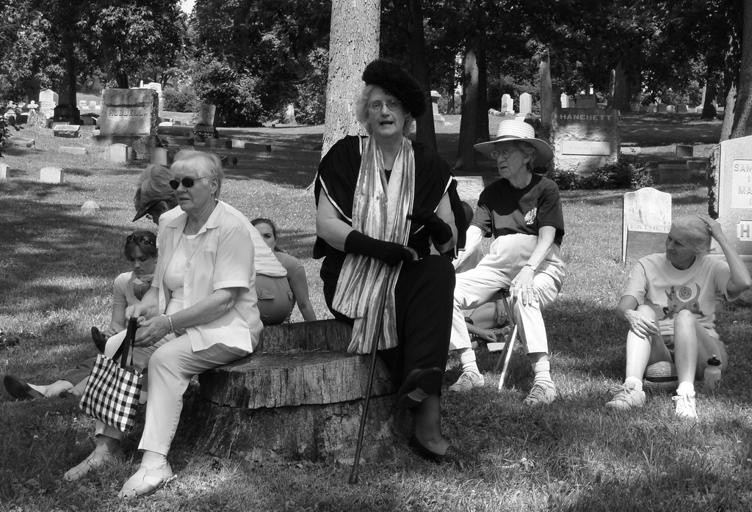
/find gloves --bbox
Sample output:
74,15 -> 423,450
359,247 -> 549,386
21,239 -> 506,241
345,230 -> 413,266
407,206 -> 453,248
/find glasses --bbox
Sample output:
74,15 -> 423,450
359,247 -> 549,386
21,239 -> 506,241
127,233 -> 157,249
367,98 -> 402,113
169,176 -> 208,189
491,147 -> 518,160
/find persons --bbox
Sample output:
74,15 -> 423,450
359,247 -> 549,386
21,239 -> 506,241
446,119 -> 567,406
312,57 -> 476,467
604,213 -> 752,419
129,163 -> 294,327
62,149 -> 265,500
3,229 -> 157,400
251,217 -> 316,323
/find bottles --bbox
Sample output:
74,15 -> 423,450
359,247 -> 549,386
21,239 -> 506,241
704,355 -> 722,394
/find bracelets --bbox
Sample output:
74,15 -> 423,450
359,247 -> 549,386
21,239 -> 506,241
159,313 -> 173,333
523,263 -> 538,272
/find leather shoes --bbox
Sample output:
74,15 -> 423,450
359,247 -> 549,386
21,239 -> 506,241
5,376 -> 47,401
397,367 -> 443,409
410,436 -> 479,468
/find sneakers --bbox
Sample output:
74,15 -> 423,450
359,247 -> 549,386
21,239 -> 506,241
605,386 -> 647,411
523,383 -> 557,409
91,327 -> 119,362
450,371 -> 484,394
672,391 -> 697,417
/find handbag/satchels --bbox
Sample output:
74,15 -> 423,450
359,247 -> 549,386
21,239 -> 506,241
78,318 -> 145,437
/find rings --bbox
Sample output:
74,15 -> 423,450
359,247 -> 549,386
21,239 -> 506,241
527,287 -> 534,290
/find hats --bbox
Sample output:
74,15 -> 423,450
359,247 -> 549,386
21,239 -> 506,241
133,199 -> 167,222
474,119 -> 554,169
362,59 -> 427,118
643,361 -> 678,392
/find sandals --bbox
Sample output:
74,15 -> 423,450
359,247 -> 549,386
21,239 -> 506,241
117,464 -> 174,499
63,442 -> 124,483
